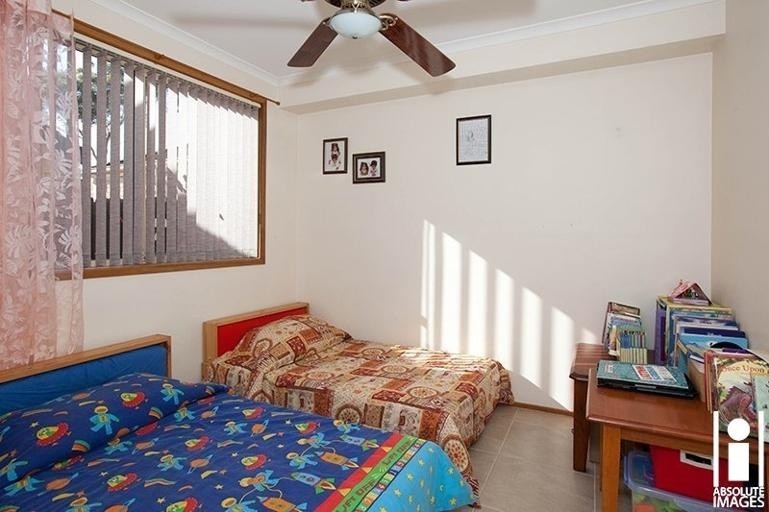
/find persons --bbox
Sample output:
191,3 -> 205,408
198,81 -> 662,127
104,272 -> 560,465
369,160 -> 377,176
359,162 -> 368,175
328,155 -> 341,170
330,143 -> 340,155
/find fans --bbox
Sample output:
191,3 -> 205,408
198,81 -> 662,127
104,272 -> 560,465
282,1 -> 458,81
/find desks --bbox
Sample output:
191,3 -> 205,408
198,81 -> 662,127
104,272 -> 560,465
586,368 -> 768,512
570,343 -> 659,474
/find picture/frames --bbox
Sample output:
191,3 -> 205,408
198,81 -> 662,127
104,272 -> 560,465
351,150 -> 387,184
455,113 -> 493,167
322,136 -> 348,175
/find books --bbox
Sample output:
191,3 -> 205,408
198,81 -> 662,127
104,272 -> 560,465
596,281 -> 769,443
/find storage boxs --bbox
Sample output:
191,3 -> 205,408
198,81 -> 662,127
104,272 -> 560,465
652,448 -> 742,506
623,451 -> 733,511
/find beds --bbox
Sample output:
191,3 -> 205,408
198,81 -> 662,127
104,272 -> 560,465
202,300 -> 519,512
0,333 -> 478,512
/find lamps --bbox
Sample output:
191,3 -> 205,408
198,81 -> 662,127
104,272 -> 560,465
318,1 -> 403,43
